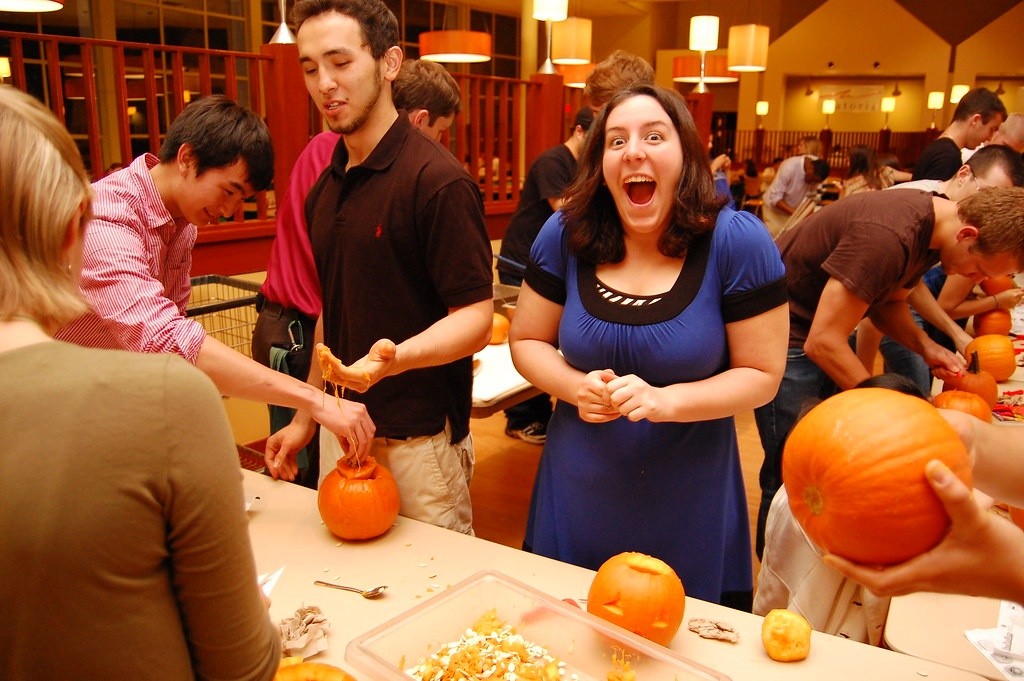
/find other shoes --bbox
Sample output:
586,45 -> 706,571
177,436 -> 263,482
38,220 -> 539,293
504,422 -> 550,444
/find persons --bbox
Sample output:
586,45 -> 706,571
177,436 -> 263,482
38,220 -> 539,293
709,147 -> 784,213
251,60 -> 465,490
763,154 -> 830,238
53,99 -> 376,467
844,144 -> 913,196
584,51 -> 655,115
753,187 -> 1024,562
752,373 -> 925,646
822,409 -> 1024,608
797,137 -> 820,156
265,1 -> 493,540
510,81 -> 789,616
856,87 -> 1024,398
0,83 -> 283,681
495,108 -> 595,444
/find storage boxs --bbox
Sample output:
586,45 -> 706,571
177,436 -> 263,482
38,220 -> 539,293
493,282 -> 521,322
344,566 -> 730,681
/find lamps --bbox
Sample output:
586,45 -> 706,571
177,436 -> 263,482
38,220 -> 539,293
892,74 -> 902,96
928,91 -> 945,130
755,100 -> 769,129
727,24 -> 768,72
821,99 -> 837,128
996,81 -> 1006,96
418,1 -> 492,63
688,12 -> 722,94
532,0 -> 599,88
879,97 -> 897,130
804,71 -> 817,97
267,0 -> 295,44
950,84 -> 971,103
670,54 -> 738,84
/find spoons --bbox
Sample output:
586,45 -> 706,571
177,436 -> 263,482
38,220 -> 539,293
314,581 -> 388,599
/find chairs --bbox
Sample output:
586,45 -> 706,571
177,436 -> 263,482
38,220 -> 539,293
741,191 -> 765,217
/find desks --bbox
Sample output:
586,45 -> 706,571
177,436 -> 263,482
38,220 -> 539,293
242,303 -> 1024,681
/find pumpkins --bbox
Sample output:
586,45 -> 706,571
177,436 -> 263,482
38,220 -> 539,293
943,349 -> 997,412
966,334 -> 1016,381
489,312 -> 510,344
929,390 -> 992,422
782,387 -> 973,566
318,453 -> 399,540
972,308 -> 1012,336
980,275 -> 1013,297
